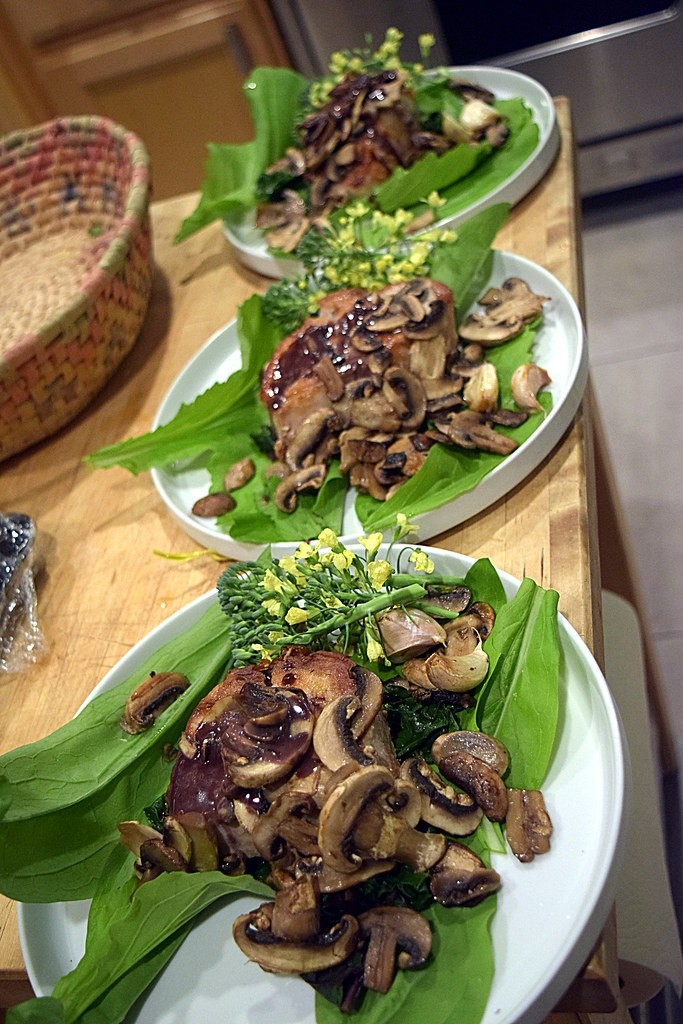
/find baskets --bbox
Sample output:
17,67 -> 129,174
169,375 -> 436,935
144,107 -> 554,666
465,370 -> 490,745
0,115 -> 156,459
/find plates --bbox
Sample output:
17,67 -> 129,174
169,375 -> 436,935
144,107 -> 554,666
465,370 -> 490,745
148,246 -> 590,563
225,66 -> 563,280
16,543 -> 631,1024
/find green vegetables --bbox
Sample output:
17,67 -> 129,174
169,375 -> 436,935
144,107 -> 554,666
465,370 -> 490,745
83,203 -> 555,544
172,26 -> 540,259
0,515 -> 562,1024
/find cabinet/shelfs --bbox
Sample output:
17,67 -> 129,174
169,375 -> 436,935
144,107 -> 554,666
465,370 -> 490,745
0,1 -> 300,204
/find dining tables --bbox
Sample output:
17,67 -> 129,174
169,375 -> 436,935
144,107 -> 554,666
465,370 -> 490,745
0,97 -> 683,1024
